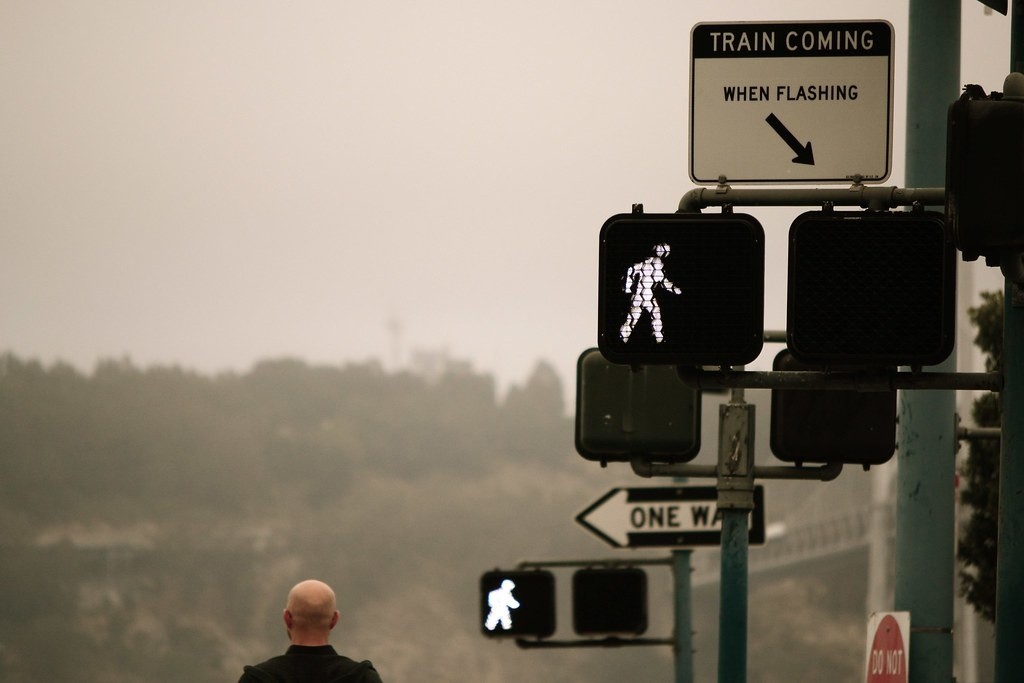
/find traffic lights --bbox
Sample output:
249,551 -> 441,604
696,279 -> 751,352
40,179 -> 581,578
572,567 -> 649,634
597,212 -> 764,368
787,210 -> 956,371
481,567 -> 557,641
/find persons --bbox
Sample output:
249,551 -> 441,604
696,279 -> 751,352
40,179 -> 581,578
237,580 -> 382,683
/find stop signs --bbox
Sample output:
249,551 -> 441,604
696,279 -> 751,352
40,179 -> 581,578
866,611 -> 911,683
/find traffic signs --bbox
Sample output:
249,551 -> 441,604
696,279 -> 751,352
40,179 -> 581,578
574,485 -> 767,548
687,20 -> 895,185
